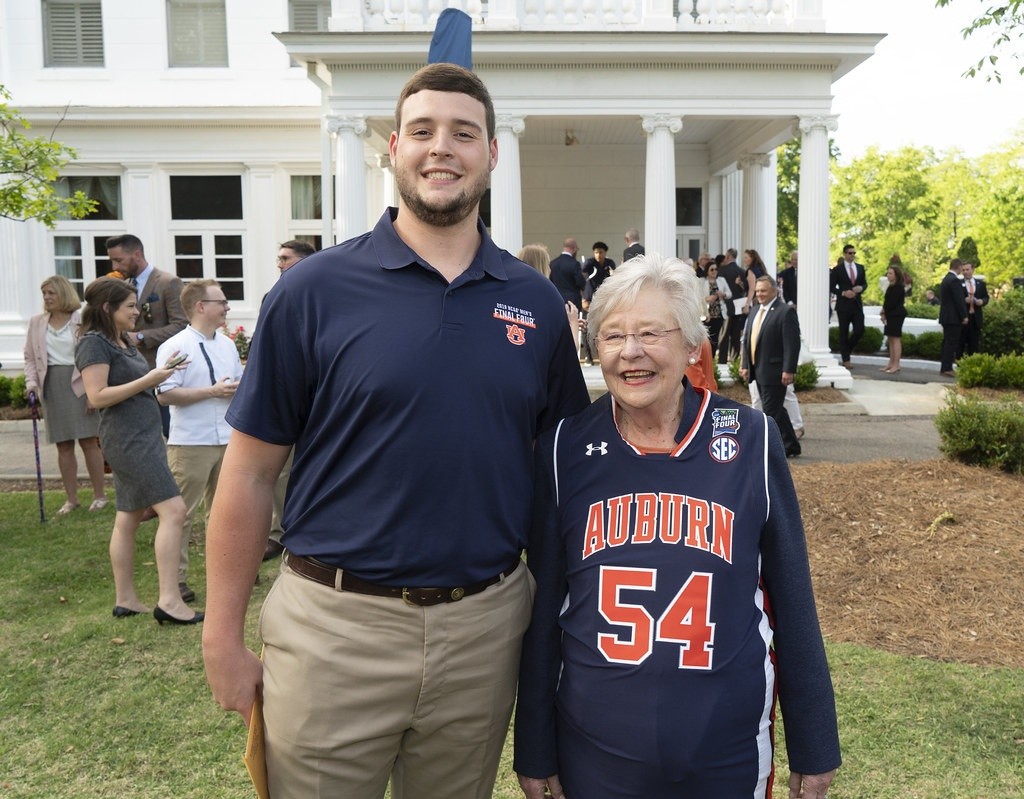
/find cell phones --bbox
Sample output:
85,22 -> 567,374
167,353 -> 189,368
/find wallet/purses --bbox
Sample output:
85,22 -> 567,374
882,320 -> 887,325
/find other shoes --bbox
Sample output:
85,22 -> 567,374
58,500 -> 80,514
843,364 -> 853,370
89,496 -> 109,512
798,428 -> 804,440
943,371 -> 954,377
262,539 -> 284,562
139,506 -> 158,522
179,582 -> 195,603
880,367 -> 900,374
785,446 -> 801,458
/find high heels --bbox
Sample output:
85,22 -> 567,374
113,606 -> 141,618
154,607 -> 204,626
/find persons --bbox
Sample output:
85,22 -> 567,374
152,278 -> 260,604
828,244 -> 868,369
517,245 -> 579,356
200,63 -> 596,799
878,266 -> 914,374
549,237 -> 616,367
927,258 -> 990,379
23,274 -> 107,516
105,234 -> 193,523
622,229 -> 645,263
514,252 -> 844,799
73,277 -> 204,625
683,248 -> 806,459
262,240 -> 316,561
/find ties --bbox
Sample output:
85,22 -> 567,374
132,280 -> 137,287
850,265 -> 854,285
968,281 -> 974,313
751,310 -> 765,365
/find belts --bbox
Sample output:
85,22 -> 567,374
288,552 -> 521,606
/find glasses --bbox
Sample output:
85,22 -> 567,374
710,269 -> 717,272
575,248 -> 579,250
848,252 -> 856,255
200,299 -> 228,304
278,255 -> 302,264
593,327 -> 681,352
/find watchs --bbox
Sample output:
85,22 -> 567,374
136,331 -> 145,346
